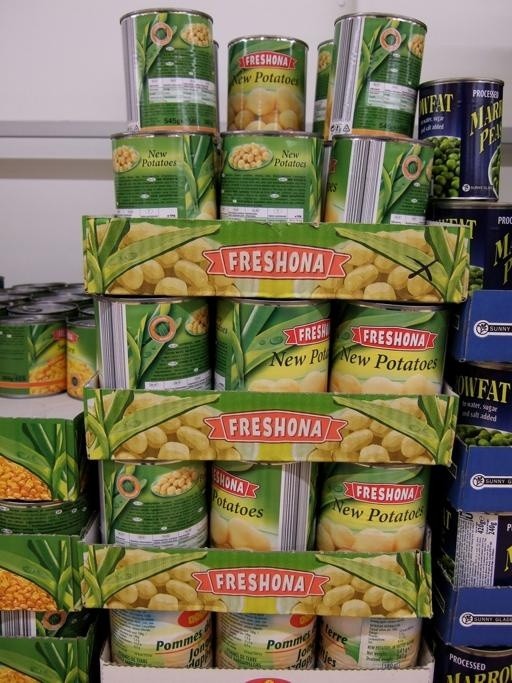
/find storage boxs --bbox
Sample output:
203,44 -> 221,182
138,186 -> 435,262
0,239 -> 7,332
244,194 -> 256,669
80,214 -> 473,305
77,509 -> 436,619
81,370 -> 459,469
433,433 -> 512,512
99,635 -> 435,683
446,290 -> 512,363
426,554 -> 512,647
0,503 -> 105,612
0,392 -> 96,503
0,622 -> 96,683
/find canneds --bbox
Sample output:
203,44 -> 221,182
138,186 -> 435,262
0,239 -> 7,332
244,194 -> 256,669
0,8 -> 512,683
